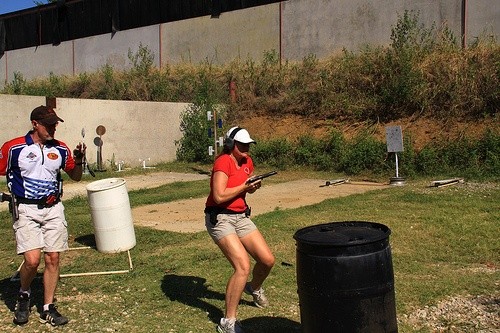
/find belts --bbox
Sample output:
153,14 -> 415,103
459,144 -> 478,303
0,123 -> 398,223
204,206 -> 245,214
16,196 -> 42,205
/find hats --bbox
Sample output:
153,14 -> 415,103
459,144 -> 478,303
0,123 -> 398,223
30,105 -> 64,125
226,127 -> 257,145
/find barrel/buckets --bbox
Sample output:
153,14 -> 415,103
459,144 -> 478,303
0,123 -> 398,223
85,177 -> 136,254
291,220 -> 398,333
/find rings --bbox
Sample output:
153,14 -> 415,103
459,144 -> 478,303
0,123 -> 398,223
255,185 -> 258,188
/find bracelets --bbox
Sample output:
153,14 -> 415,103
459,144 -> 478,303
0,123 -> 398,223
74,161 -> 82,166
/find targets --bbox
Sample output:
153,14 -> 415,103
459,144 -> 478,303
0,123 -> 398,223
206,110 -> 224,156
384,125 -> 406,184
93,125 -> 108,173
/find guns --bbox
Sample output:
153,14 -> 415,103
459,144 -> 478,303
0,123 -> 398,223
8,194 -> 20,221
247,170 -> 278,183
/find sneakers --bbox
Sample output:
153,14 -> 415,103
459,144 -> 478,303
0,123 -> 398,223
217,317 -> 246,332
13,292 -> 32,324
243,281 -> 270,309
39,304 -> 68,326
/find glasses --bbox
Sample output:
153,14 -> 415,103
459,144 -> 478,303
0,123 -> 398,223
37,121 -> 59,126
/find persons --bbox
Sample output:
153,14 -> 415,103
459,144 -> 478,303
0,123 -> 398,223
204,127 -> 275,333
0,105 -> 88,326
